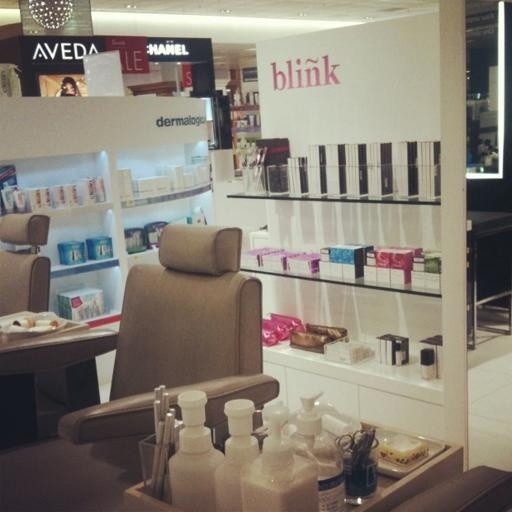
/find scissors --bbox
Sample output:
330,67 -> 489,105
336,429 -> 379,456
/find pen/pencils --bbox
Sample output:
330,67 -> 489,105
148,384 -> 175,496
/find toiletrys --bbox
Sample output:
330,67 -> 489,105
165,386 -> 345,512
1,176 -> 106,213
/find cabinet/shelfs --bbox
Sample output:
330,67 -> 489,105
226,194 -> 440,389
0,95 -> 216,385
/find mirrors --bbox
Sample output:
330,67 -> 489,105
466,1 -> 504,180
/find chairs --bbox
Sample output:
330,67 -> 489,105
1,222 -> 279,512
2,214 -> 50,312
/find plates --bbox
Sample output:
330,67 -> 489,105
1,317 -> 69,335
